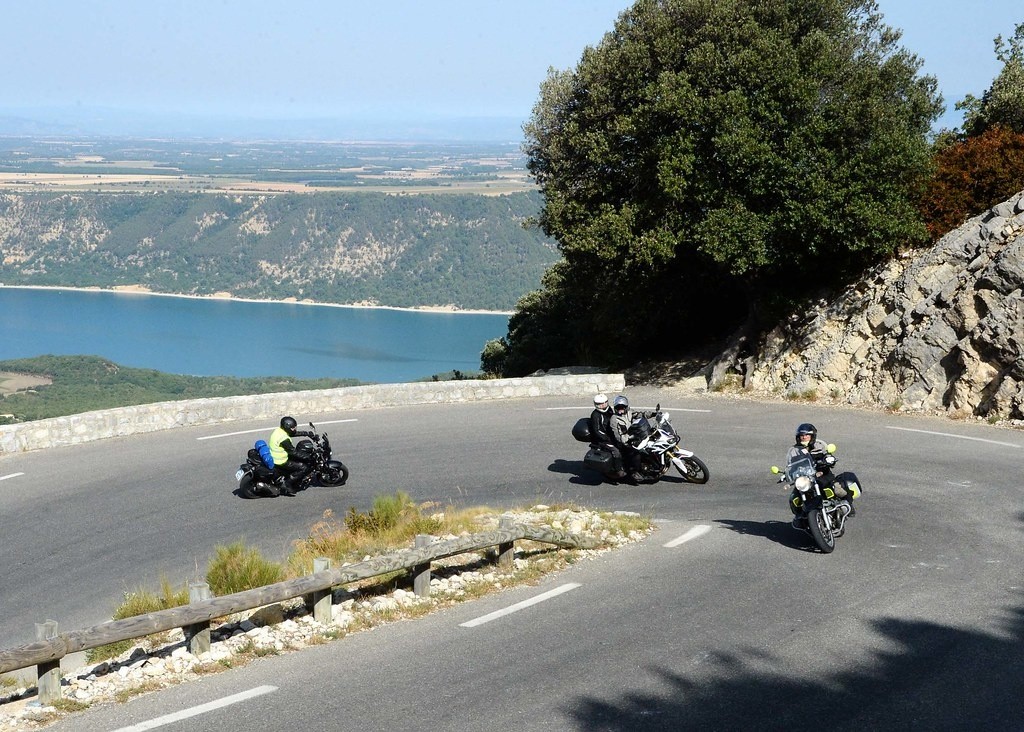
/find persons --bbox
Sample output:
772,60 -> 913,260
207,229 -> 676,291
268,416 -> 314,494
590,394 -> 627,477
609,393 -> 662,482
780,423 -> 856,530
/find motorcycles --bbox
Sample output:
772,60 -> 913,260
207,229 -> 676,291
771,444 -> 862,554
236,421 -> 349,499
571,403 -> 710,485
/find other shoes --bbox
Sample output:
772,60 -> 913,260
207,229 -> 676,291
847,505 -> 855,517
632,473 -> 645,481
280,479 -> 296,493
617,471 -> 627,477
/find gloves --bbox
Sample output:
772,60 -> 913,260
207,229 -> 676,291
653,412 -> 663,416
308,431 -> 315,437
826,456 -> 835,465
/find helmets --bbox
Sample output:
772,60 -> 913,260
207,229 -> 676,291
280,416 -> 298,436
795,423 -> 818,446
593,394 -> 609,413
613,395 -> 628,416
